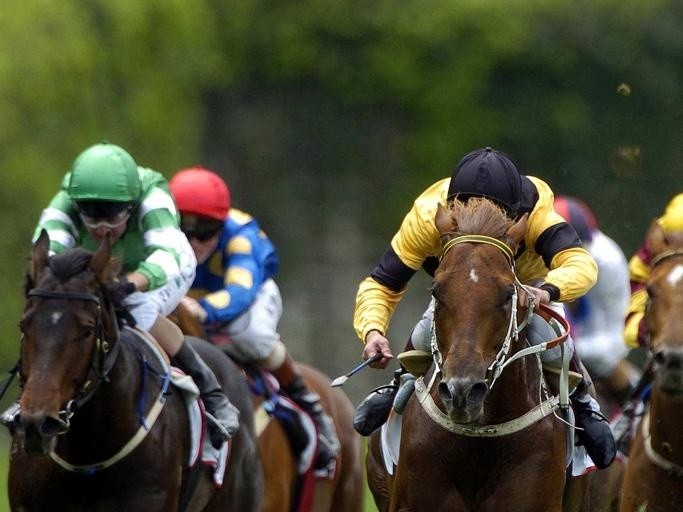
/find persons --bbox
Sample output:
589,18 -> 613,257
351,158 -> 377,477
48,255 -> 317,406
622,188 -> 682,434
349,144 -> 617,469
166,160 -> 342,476
1,137 -> 243,446
542,192 -> 641,413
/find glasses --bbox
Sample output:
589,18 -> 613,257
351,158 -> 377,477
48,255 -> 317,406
180,220 -> 225,244
74,201 -> 140,229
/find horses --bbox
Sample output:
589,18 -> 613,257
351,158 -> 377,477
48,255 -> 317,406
236,357 -> 364,512
615,220 -> 682,512
5,225 -> 266,512
363,194 -> 579,512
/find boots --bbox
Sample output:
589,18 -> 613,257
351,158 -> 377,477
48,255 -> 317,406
279,364 -> 343,472
168,334 -> 243,447
351,327 -> 418,439
568,344 -> 620,471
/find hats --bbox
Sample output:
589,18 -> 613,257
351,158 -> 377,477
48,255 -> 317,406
168,163 -> 232,222
445,145 -> 523,218
552,194 -> 598,243
66,138 -> 144,204
